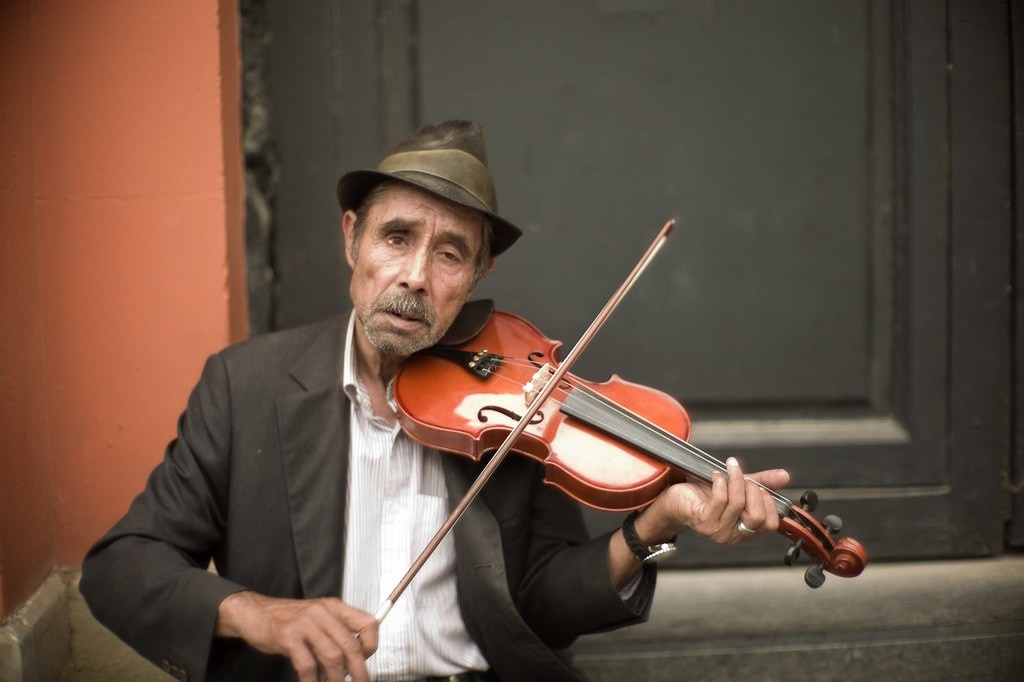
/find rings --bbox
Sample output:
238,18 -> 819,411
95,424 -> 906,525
737,520 -> 756,537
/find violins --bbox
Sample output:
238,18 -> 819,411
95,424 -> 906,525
385,298 -> 869,589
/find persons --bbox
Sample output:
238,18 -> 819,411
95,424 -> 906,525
79,123 -> 790,682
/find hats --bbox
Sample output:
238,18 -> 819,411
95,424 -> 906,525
337,120 -> 523,259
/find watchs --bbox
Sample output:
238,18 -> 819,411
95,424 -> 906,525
622,511 -> 679,563
